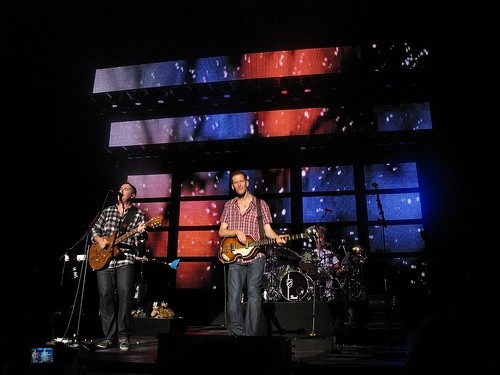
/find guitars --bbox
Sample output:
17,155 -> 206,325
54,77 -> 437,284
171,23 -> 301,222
87,213 -> 165,271
217,228 -> 321,265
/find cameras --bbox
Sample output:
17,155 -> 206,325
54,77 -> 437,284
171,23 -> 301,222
29,345 -> 56,369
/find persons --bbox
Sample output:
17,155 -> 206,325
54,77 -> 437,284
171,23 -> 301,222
303,237 -> 342,301
32,348 -> 49,363
219,171 -> 290,336
90,181 -> 149,350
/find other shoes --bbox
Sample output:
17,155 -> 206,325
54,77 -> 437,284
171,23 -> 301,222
232,328 -> 245,336
97,339 -> 115,349
119,337 -> 130,350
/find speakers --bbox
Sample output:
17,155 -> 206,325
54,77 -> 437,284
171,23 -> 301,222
157,333 -> 292,366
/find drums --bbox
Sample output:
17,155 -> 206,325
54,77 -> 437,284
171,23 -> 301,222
258,270 -> 280,299
278,270 -> 314,302
299,249 -> 319,272
316,251 -> 334,267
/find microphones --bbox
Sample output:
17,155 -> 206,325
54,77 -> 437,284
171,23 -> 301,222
110,190 -> 123,196
325,209 -> 332,212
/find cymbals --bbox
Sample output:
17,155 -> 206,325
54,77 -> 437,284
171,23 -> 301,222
273,247 -> 302,260
316,243 -> 331,248
306,226 -> 327,234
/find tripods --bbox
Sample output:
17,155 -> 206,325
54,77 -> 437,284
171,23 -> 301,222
59,192 -> 109,352
